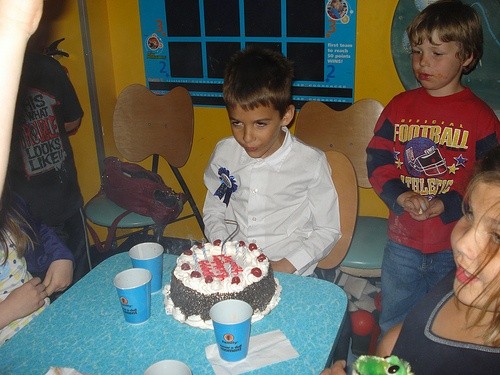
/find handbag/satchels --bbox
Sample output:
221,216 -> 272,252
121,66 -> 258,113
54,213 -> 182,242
101,156 -> 184,223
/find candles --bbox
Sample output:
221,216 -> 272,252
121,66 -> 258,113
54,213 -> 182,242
192,239 -> 247,279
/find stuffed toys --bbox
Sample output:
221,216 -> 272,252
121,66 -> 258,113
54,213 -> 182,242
351,354 -> 414,375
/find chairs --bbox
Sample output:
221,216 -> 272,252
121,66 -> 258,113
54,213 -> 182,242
293,99 -> 390,278
85,83 -> 208,253
314,152 -> 359,281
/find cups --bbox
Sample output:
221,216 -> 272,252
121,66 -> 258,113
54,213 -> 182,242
129,242 -> 165,295
209,299 -> 253,363
113,268 -> 152,325
144,360 -> 192,375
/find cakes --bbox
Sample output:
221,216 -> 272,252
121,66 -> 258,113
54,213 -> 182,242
169,239 -> 277,322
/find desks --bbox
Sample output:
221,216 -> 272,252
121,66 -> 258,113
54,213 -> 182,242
0,252 -> 350,375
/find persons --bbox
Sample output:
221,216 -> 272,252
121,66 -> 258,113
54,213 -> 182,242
0,171 -> 74,348
365,0 -> 500,347
202,45 -> 343,277
317,146 -> 500,375
0,0 -> 44,197
8,44 -> 88,305
